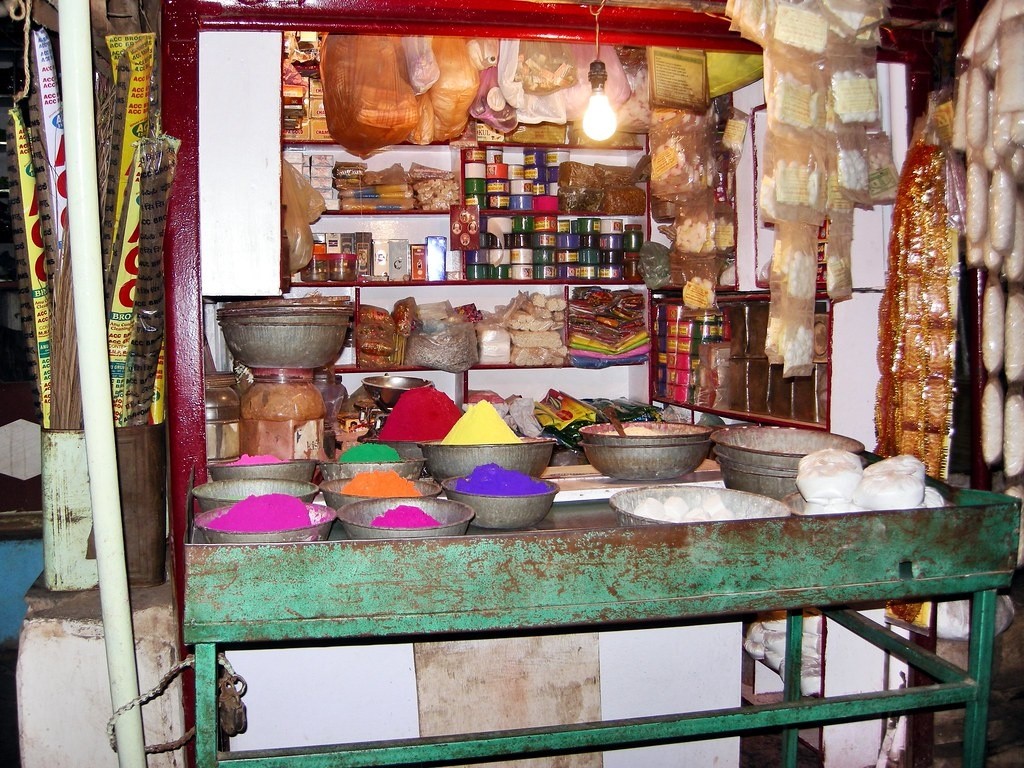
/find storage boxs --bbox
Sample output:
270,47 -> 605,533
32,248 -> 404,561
283,152 -> 340,211
310,233 -> 447,281
281,75 -> 335,140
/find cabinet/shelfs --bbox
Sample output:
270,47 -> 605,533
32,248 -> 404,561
275,31 -> 835,431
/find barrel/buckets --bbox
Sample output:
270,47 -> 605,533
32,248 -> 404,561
40,428 -> 99,592
115,422 -> 167,587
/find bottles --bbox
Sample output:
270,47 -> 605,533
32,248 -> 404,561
623,223 -> 644,252
623,251 -> 642,279
205,368 -> 348,477
302,253 -> 357,282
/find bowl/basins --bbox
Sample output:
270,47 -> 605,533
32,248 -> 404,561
356,435 -> 423,459
577,439 -> 713,481
362,375 -> 435,408
610,483 -> 792,526
192,477 -> 320,511
318,475 -> 442,508
193,502 -> 338,543
416,437 -> 559,486
205,457 -> 318,482
440,474 -> 561,529
708,424 -> 866,500
779,483 -> 946,516
578,421 -> 713,446
320,453 -> 430,481
336,497 -> 476,540
